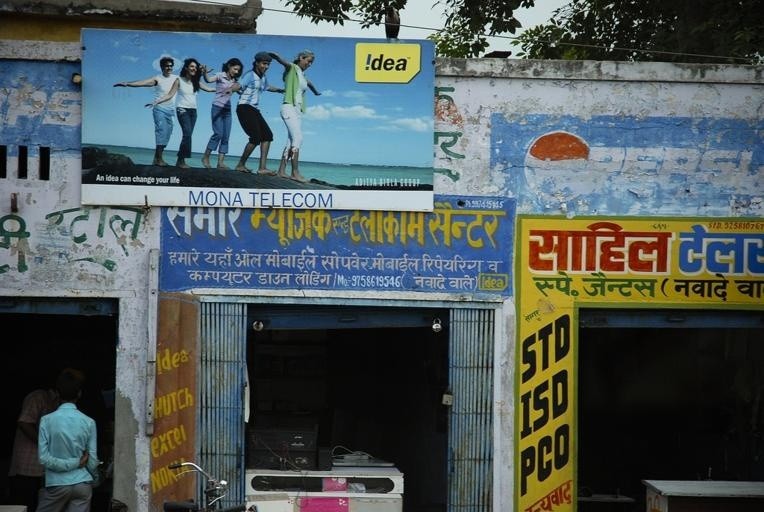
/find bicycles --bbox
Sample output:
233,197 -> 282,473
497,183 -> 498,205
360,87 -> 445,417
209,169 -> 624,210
162,462 -> 230,511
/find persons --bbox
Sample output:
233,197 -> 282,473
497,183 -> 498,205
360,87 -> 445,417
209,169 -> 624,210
8,372 -> 61,512
111,49 -> 322,184
36,367 -> 99,512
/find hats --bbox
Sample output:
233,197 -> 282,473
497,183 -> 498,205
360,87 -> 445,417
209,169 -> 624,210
255,51 -> 273,62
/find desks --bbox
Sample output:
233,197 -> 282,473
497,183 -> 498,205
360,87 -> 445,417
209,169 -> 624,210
639,479 -> 764,511
